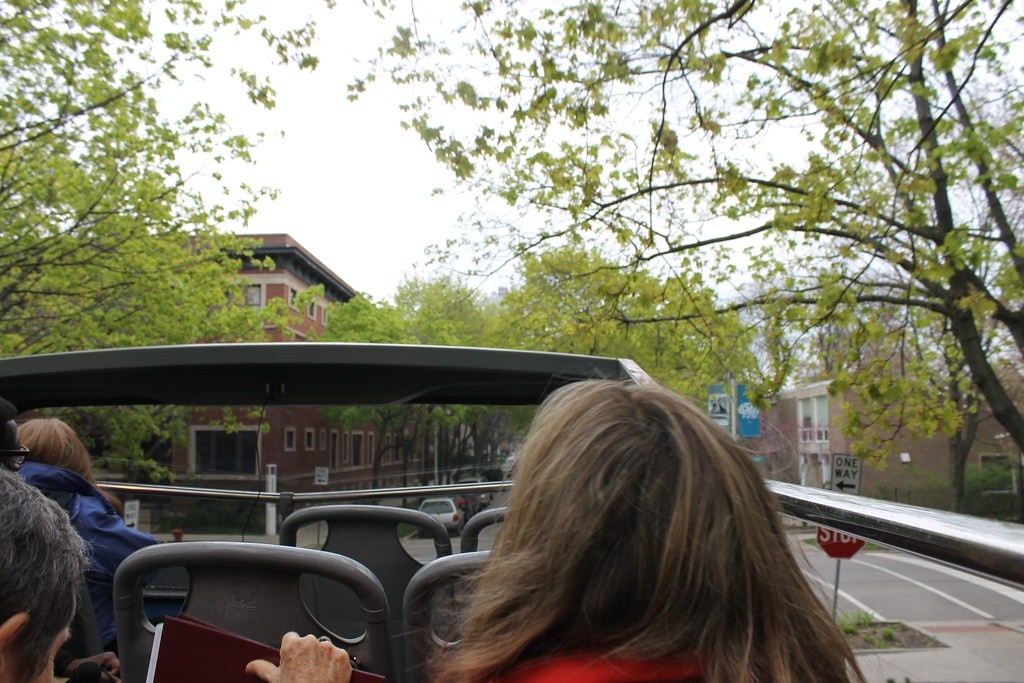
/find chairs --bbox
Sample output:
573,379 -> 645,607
55,503 -> 510,683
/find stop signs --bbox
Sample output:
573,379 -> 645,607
816,526 -> 864,560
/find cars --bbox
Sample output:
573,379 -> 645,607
418,498 -> 464,539
449,456 -> 515,520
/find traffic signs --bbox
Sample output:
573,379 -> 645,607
123,499 -> 141,530
313,466 -> 330,486
830,451 -> 861,497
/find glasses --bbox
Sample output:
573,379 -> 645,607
0,445 -> 30,464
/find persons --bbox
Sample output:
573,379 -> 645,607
0,397 -> 352,683
424,379 -> 864,683
